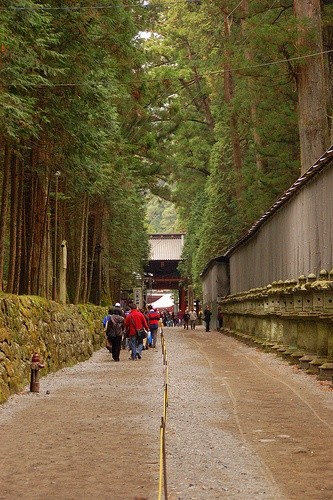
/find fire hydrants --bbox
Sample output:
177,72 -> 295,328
29,353 -> 44,393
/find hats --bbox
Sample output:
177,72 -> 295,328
115,302 -> 120,306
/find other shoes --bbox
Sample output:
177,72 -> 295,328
136,351 -> 141,359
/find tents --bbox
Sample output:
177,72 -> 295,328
151,294 -> 174,309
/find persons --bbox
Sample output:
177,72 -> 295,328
103,302 -> 160,362
189,308 -> 197,330
182,310 -> 190,330
198,309 -> 203,324
217,306 -> 224,331
202,305 -> 212,332
159,309 -> 177,328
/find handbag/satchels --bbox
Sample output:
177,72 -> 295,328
106,336 -> 112,352
150,324 -> 158,330
109,328 -> 116,338
136,328 -> 148,340
